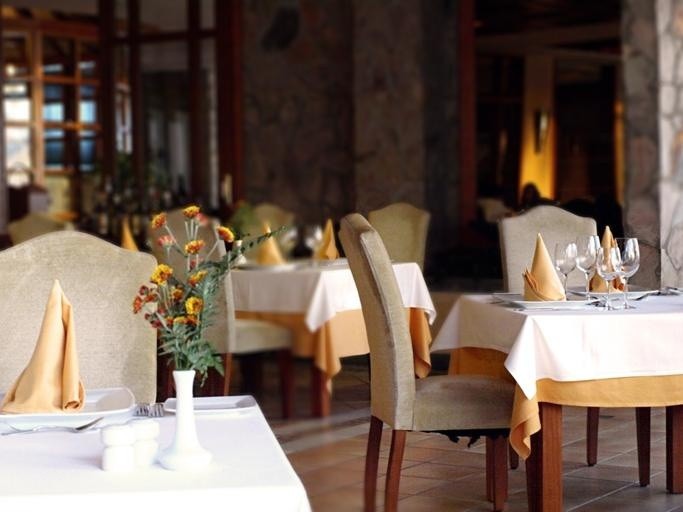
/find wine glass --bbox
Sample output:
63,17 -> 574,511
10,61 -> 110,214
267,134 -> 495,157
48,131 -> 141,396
303,225 -> 326,267
597,246 -> 621,311
555,240 -> 577,301
575,235 -> 601,303
277,226 -> 298,264
612,237 -> 641,310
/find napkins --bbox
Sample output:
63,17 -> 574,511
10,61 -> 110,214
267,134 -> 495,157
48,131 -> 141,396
590,226 -> 626,292
524,232 -> 568,299
4,278 -> 86,412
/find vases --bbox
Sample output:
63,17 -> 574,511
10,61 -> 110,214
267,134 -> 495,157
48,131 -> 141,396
158,369 -> 208,471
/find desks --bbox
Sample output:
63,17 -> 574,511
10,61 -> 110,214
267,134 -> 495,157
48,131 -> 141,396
2,396 -> 309,512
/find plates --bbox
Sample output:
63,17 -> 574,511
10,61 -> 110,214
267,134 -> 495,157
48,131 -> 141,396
570,290 -> 660,301
162,395 -> 255,412
0,386 -> 134,431
493,292 -> 599,310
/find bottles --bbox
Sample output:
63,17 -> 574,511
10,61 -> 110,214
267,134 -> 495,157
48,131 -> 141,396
93,170 -> 188,239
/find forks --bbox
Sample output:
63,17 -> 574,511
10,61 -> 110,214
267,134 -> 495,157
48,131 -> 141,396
135,402 -> 163,418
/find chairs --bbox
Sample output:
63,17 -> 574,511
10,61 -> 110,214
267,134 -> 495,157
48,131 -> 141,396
1,204 -> 150,249
500,208 -> 597,293
0,230 -> 157,405
124,202 -> 437,416
338,212 -> 520,511
